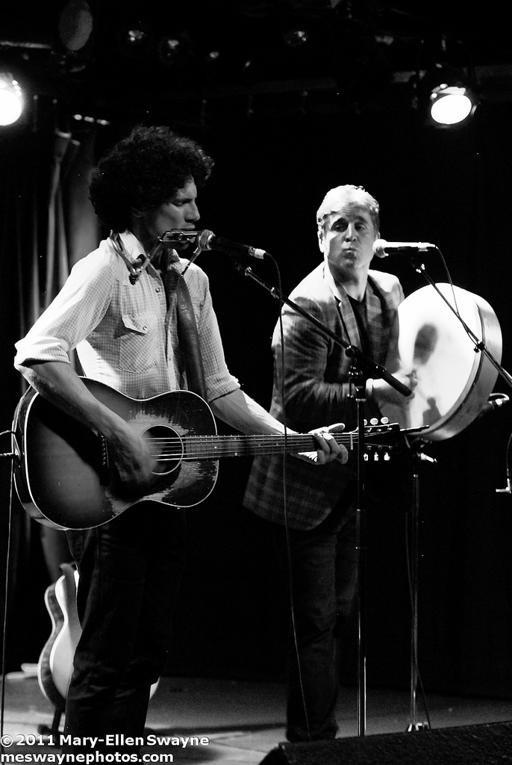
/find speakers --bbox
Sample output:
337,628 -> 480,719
258,720 -> 512,765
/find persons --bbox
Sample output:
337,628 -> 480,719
244,180 -> 411,742
10,126 -> 349,762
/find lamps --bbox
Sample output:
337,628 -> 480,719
419,62 -> 480,136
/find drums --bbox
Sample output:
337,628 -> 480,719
379,282 -> 503,440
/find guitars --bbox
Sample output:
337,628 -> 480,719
12,375 -> 400,532
37,530 -> 159,709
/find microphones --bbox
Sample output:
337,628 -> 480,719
372,239 -> 437,259
197,229 -> 267,261
